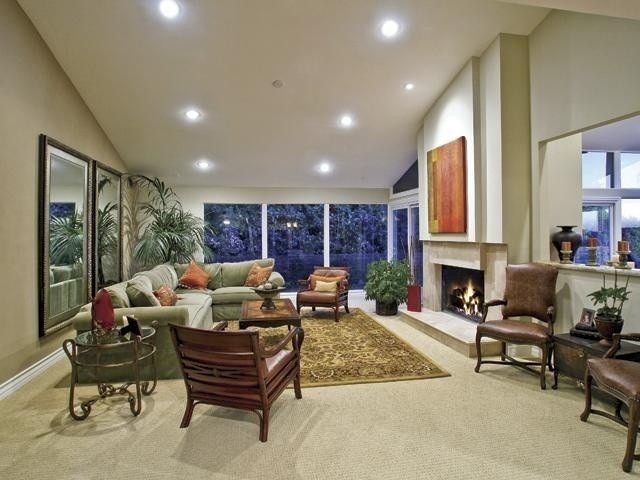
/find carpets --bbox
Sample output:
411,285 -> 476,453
211,306 -> 452,389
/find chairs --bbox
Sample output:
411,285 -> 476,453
475,262 -> 558,390
167,318 -> 306,443
296,264 -> 352,323
580,332 -> 640,474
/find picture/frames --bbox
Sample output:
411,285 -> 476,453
579,308 -> 596,327
118,315 -> 142,337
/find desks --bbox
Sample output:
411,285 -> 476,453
550,333 -> 640,421
62,324 -> 158,422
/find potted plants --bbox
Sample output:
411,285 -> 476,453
50,172 -> 119,292
585,269 -> 632,348
363,259 -> 414,316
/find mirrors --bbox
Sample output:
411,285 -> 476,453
37,134 -> 94,342
95,160 -> 123,300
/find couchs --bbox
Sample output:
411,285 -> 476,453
70,257 -> 286,386
49,262 -> 83,317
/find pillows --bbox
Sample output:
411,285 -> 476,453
313,270 -> 348,289
152,283 -> 178,306
309,274 -> 346,291
177,260 -> 211,290
126,281 -> 162,307
241,263 -> 274,287
314,280 -> 338,292
